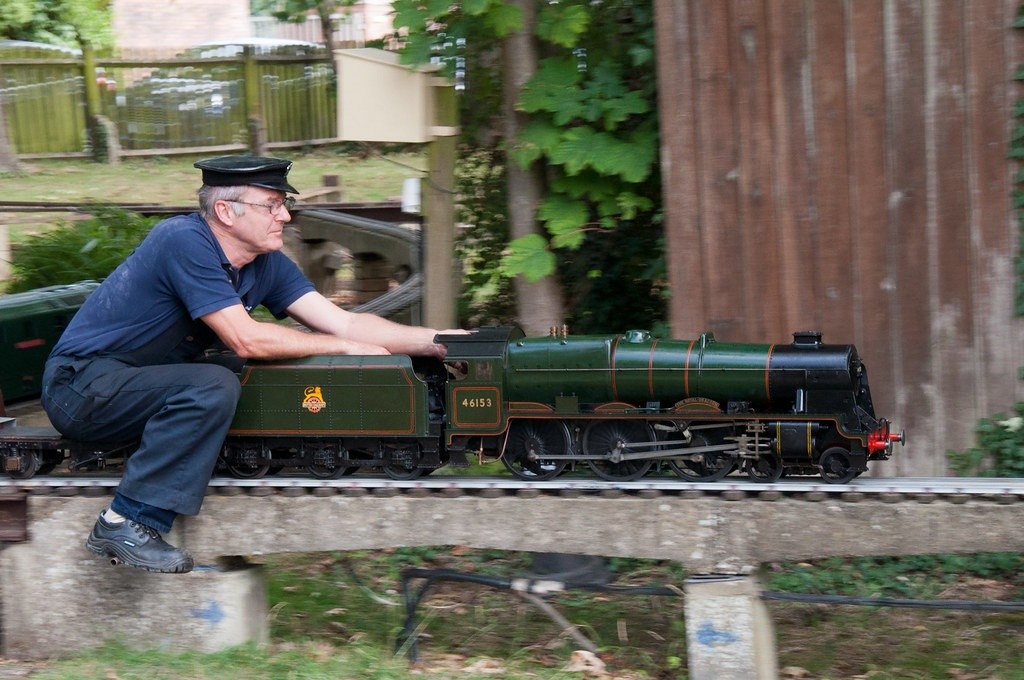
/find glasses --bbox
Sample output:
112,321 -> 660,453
224,197 -> 296,216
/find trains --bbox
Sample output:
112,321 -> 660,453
0,281 -> 907,489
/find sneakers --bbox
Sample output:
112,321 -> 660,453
86,509 -> 194,574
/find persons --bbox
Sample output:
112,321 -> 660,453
38,154 -> 479,573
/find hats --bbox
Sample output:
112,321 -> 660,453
194,155 -> 300,195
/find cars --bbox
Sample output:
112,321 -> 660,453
120,40 -> 337,148
0,37 -> 117,138
